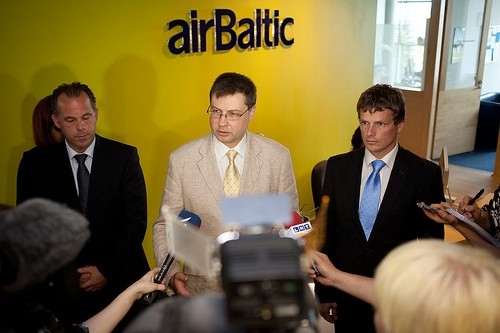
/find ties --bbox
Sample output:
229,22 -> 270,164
74,154 -> 91,215
357,160 -> 386,243
222,149 -> 242,200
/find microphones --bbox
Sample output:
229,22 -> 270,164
284,211 -> 322,277
139,209 -> 201,305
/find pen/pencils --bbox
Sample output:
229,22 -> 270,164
461,188 -> 485,214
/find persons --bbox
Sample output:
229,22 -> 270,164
306,238 -> 500,332
32,81 -> 150,323
15,94 -> 66,211
1,269 -> 167,333
153,66 -> 305,299
420,182 -> 500,260
309,84 -> 446,333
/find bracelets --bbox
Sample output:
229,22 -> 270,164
475,210 -> 483,221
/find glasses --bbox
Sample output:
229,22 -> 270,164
207,105 -> 253,121
